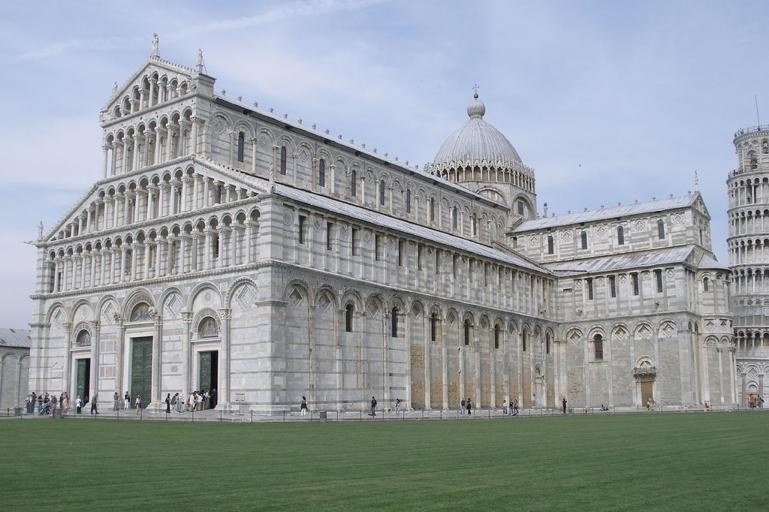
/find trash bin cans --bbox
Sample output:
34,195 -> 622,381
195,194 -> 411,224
15,408 -> 21,416
569,409 -> 572,414
320,410 -> 327,422
56,409 -> 62,416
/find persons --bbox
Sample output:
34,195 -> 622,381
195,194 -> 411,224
370,396 -> 377,416
395,397 -> 401,416
300,395 -> 307,416
502,399 -> 508,415
123,391 -> 130,411
562,397 -> 568,414
513,399 -> 519,414
466,397 -> 473,414
113,391 -> 119,411
460,396 -> 466,415
509,399 -> 514,416
25,392 -> 70,415
90,393 -> 100,414
135,394 -> 142,415
75,394 -> 83,414
83,395 -> 89,406
165,388 -> 218,414
